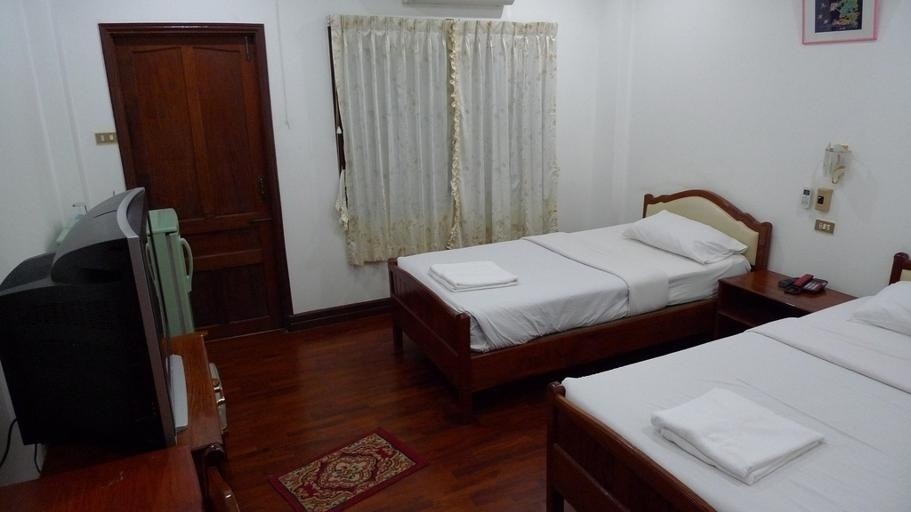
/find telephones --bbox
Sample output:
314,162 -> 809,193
794,274 -> 828,293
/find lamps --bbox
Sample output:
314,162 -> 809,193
823,142 -> 852,184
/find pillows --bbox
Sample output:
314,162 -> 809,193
854,279 -> 910,337
622,209 -> 749,266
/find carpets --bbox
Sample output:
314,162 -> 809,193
267,425 -> 428,512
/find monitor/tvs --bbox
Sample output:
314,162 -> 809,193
0,187 -> 189,450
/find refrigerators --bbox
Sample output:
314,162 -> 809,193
56,207 -> 195,339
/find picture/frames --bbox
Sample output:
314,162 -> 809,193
802,0 -> 879,45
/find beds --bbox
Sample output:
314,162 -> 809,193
546,253 -> 910,512
386,189 -> 773,426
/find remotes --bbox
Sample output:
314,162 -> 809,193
779,278 -> 800,288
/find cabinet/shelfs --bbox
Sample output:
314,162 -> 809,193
712,269 -> 859,341
1,331 -> 239,511
